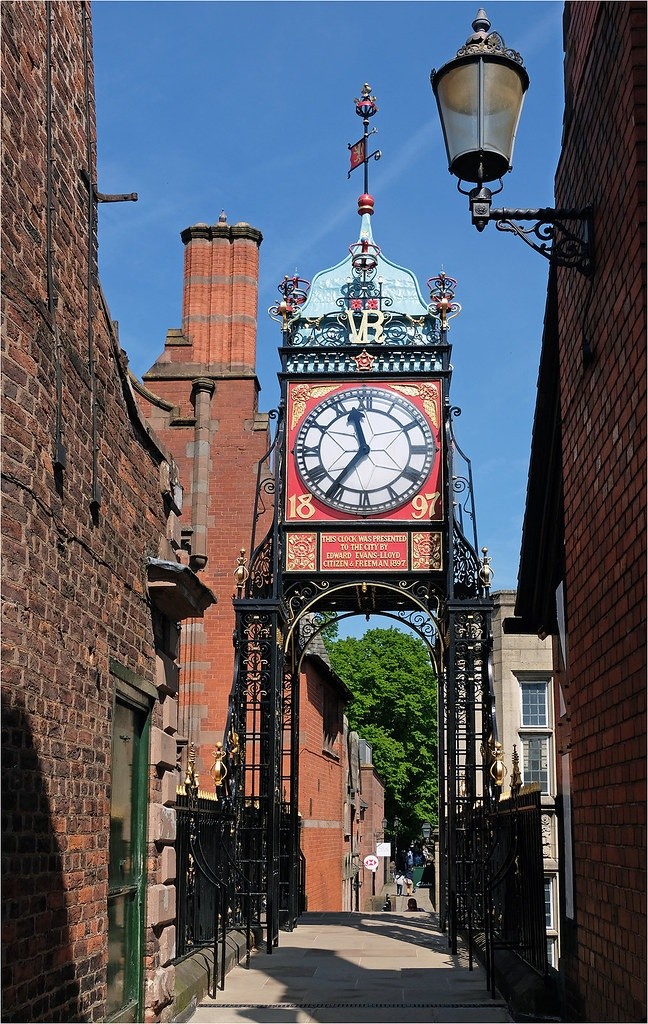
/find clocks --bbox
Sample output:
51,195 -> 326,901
295,385 -> 436,516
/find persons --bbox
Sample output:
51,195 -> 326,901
395,869 -> 414,896
404,898 -> 426,912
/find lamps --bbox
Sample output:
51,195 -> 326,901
374,814 -> 401,839
421,819 -> 439,857
428,6 -> 602,280
350,881 -> 363,891
426,860 -> 435,866
